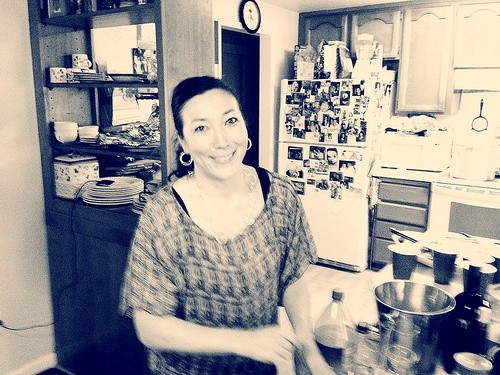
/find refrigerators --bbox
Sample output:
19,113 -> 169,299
276,71 -> 396,273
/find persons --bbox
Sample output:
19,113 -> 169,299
117,76 -> 338,375
285,79 -> 391,200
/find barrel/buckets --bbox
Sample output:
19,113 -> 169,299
374,280 -> 456,375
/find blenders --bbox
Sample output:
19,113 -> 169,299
352,34 -> 375,79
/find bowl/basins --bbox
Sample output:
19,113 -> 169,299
54,121 -> 99,143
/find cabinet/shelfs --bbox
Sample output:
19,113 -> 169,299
394,0 -> 456,115
368,167 -> 441,274
297,2 -> 402,61
28,0 -> 216,375
453,0 -> 500,94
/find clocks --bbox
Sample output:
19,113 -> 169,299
239,0 -> 262,34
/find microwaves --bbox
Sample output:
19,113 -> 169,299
381,126 -> 454,173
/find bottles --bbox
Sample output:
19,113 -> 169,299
40,0 -> 153,19
311,287 -> 348,370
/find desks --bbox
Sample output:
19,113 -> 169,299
343,262 -> 465,327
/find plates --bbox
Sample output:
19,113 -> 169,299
83,176 -> 155,215
72,71 -> 148,83
389,229 -> 500,272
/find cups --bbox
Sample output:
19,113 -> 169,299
387,243 -> 421,281
352,321 -> 492,375
49,67 -> 96,84
71,53 -> 92,70
455,256 -> 500,329
432,247 -> 457,286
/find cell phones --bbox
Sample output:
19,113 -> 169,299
96,180 -> 114,186
